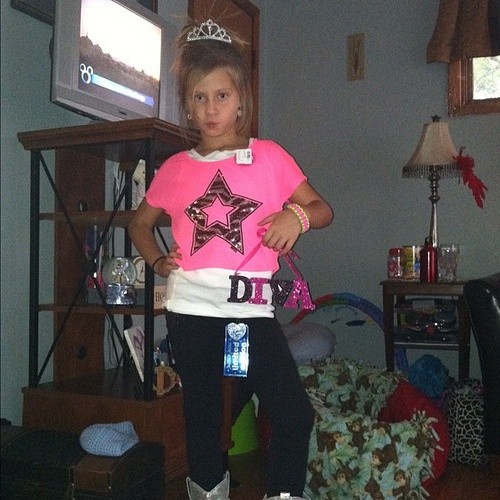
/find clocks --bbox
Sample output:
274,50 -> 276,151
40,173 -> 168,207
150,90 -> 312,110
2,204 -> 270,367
130,255 -> 145,289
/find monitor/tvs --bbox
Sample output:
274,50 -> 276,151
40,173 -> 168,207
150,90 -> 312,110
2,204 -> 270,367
50,0 -> 177,124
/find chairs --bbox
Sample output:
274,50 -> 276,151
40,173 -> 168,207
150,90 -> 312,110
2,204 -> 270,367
464,273 -> 500,454
260,360 -> 449,500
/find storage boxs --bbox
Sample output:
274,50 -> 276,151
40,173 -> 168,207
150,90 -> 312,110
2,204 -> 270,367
0,429 -> 166,500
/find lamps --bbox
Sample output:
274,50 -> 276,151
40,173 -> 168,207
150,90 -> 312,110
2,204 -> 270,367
402,115 -> 462,249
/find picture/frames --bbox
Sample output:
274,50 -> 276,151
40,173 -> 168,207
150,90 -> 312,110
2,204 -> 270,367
124,326 -> 144,383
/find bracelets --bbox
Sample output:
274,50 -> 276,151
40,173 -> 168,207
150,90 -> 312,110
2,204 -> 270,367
152,256 -> 166,273
286,202 -> 310,233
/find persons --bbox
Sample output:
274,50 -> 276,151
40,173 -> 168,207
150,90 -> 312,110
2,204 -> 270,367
128,15 -> 333,499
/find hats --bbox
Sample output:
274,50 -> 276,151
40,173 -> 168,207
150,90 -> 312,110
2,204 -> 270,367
80,419 -> 139,457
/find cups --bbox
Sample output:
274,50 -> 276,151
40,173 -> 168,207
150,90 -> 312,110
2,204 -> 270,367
438,242 -> 460,284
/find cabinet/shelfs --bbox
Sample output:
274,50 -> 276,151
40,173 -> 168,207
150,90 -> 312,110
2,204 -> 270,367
379,279 -> 470,380
17,117 -> 233,479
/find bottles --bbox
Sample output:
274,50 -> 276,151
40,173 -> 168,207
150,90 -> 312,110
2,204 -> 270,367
420,237 -> 438,285
387,248 -> 404,280
